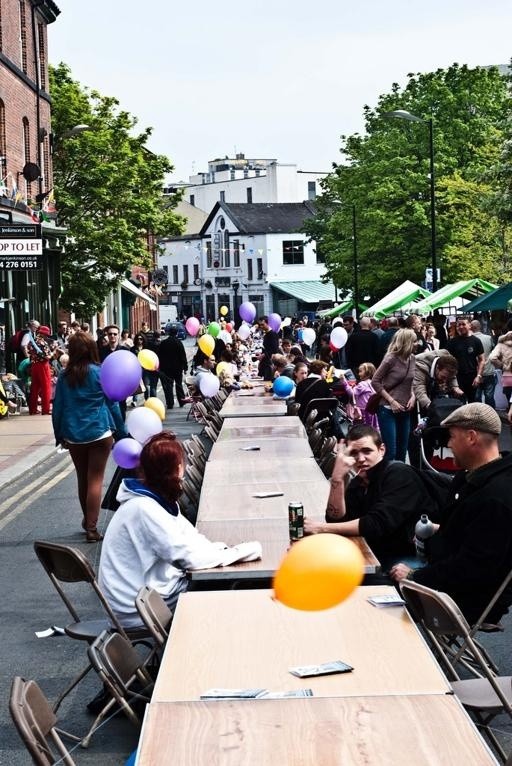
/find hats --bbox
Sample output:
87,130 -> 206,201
439,403 -> 503,435
38,325 -> 51,337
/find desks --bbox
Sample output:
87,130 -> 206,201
150,584 -> 453,703
184,413 -> 382,584
133,694 -> 500,765
217,377 -> 287,417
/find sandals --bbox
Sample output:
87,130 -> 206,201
86,528 -> 103,542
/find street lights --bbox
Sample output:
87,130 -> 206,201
332,199 -> 364,316
393,108 -> 444,293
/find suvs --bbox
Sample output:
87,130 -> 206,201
291,311 -> 325,326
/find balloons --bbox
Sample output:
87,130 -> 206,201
198,334 -> 216,357
200,374 -> 219,398
268,314 -> 282,333
208,322 -> 220,337
238,325 -> 250,340
112,438 -> 144,469
138,348 -> 160,372
100,350 -> 142,402
216,361 -> 232,377
302,328 -> 316,349
270,533 -> 365,610
186,317 -> 200,337
331,327 -> 348,349
144,397 -> 166,420
239,302 -> 256,323
219,305 -> 229,316
298,330 -> 303,342
272,376 -> 294,397
226,323 -> 232,333
127,407 -> 163,443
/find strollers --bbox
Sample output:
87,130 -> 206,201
414,390 -> 470,482
324,364 -> 356,430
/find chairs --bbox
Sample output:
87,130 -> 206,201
10,675 -> 76,766
134,583 -> 173,650
398,578 -> 511,764
31,540 -> 154,715
183,375 -> 228,428
87,629 -> 154,725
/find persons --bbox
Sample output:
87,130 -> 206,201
52,329 -> 129,542
190,316 -> 309,409
1,319 -> 102,415
96,430 -> 261,639
291,313 -> 512,464
96,322 -> 189,409
362,402 -> 511,625
303,425 -> 435,565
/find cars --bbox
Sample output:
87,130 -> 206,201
165,322 -> 187,340
